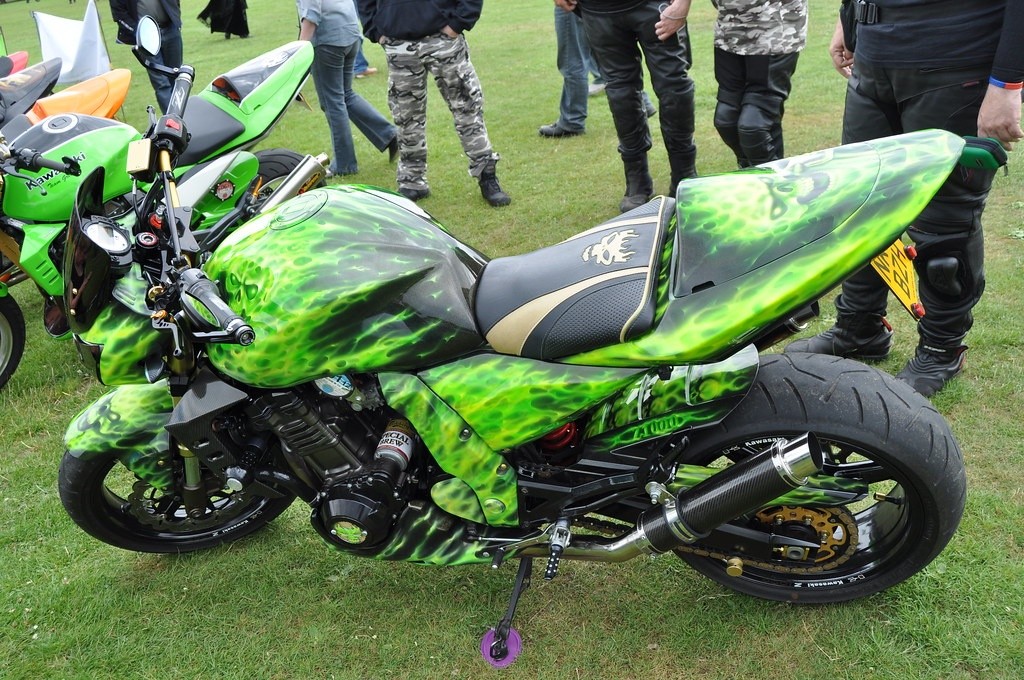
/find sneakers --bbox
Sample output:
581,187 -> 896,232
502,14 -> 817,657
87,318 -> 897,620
538,123 -> 579,137
355,68 -> 378,78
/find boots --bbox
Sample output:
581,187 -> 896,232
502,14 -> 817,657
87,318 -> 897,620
619,151 -> 653,213
478,164 -> 512,207
668,145 -> 700,199
397,188 -> 432,201
783,294 -> 894,363
895,321 -> 969,398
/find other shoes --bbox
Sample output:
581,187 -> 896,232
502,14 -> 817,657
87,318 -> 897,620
325,168 -> 358,178
389,135 -> 400,163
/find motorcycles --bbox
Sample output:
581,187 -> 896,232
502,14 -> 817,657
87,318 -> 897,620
0,47 -> 134,145
53,10 -> 1012,667
0,38 -> 316,394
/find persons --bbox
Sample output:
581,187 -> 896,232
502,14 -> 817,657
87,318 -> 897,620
353,50 -> 378,78
355,0 -> 512,208
712,0 -> 809,167
538,0 -> 656,136
109,0 -> 183,114
554,0 -> 696,213
782,0 -> 1024,399
296,0 -> 397,178
196,0 -> 250,39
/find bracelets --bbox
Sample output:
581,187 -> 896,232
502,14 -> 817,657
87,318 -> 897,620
989,76 -> 1023,90
